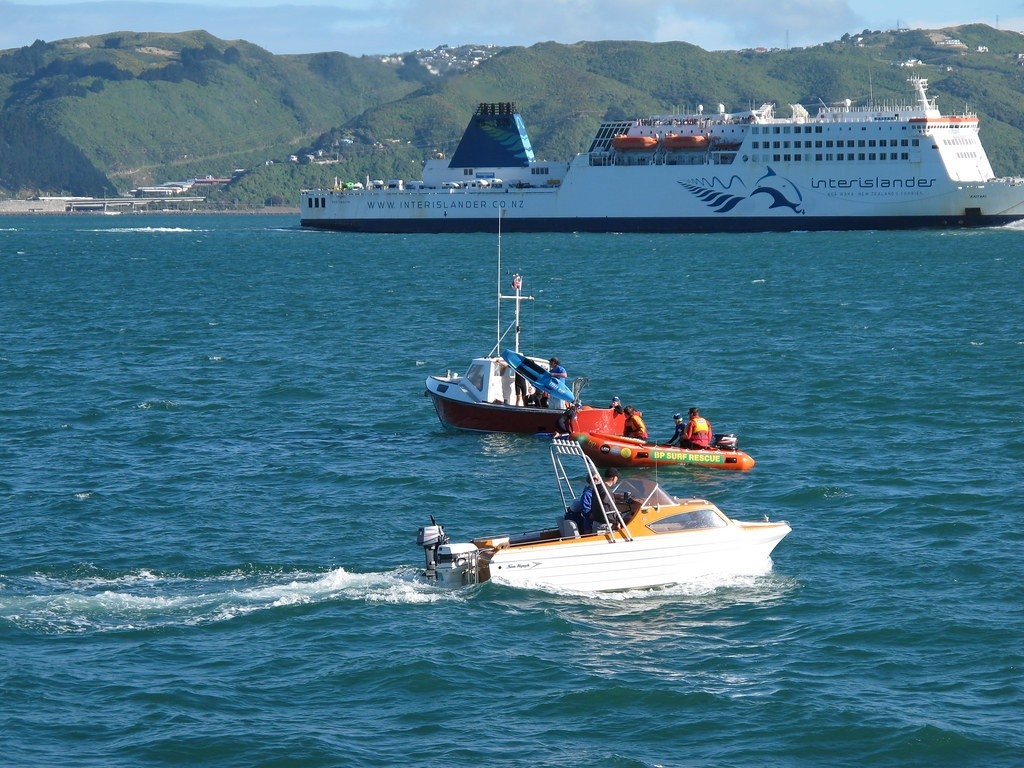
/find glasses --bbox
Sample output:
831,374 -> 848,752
688,412 -> 692,415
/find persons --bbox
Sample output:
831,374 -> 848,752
591,467 -> 620,535
664,413 -> 687,448
609,396 -> 622,415
623,405 -> 648,441
528,385 -> 549,409
579,471 -> 600,536
551,405 -> 579,439
493,359 -> 528,407
681,407 -> 712,451
548,357 -> 567,410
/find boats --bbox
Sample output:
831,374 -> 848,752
571,430 -> 756,471
426,205 -> 641,439
302,74 -> 1024,233
416,439 -> 793,594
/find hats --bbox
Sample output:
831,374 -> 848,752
548,356 -> 559,364
587,471 -> 596,483
612,396 -> 620,401
674,413 -> 682,419
605,467 -> 622,476
